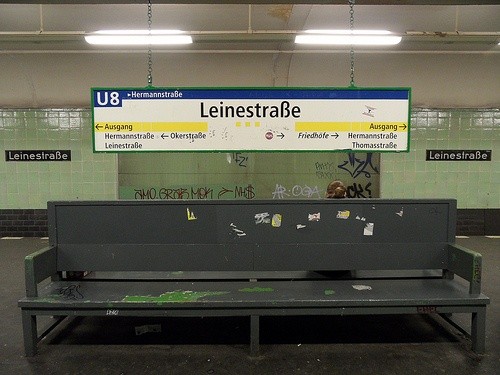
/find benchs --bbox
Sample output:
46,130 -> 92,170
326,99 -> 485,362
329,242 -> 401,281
17,199 -> 490,362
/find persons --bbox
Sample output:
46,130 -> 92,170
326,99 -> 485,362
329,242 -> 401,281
327,181 -> 346,198
